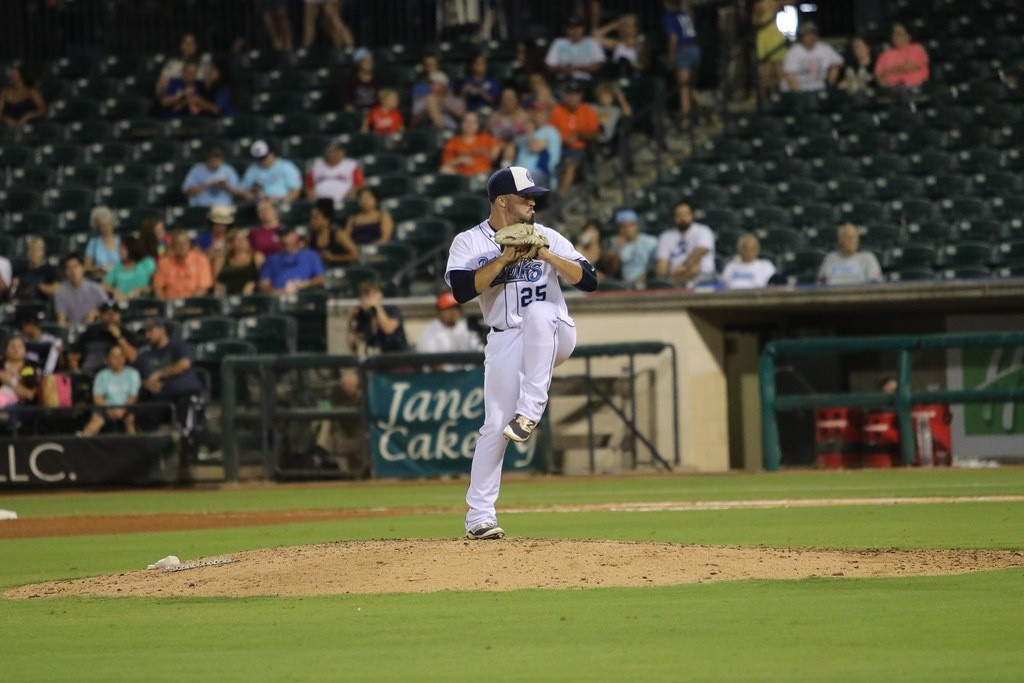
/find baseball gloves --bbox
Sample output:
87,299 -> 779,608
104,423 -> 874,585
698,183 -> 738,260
494,221 -> 551,261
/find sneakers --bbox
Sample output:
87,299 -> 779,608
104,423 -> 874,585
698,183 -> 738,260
503,414 -> 538,442
467,523 -> 505,540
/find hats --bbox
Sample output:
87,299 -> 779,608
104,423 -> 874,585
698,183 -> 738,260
206,205 -> 235,223
99,300 -> 119,314
617,209 -> 637,223
487,166 -> 550,199
798,23 -> 816,35
250,139 -> 269,158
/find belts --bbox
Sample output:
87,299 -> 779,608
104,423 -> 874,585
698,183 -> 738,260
492,326 -> 504,332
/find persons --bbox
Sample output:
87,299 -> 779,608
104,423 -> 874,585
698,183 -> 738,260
751,0 -> 928,107
0,140 -> 411,437
341,0 -> 705,193
154,32 -> 220,119
416,292 -> 485,374
0,67 -> 47,126
559,205 -> 788,291
816,223 -> 881,286
444,167 -> 598,542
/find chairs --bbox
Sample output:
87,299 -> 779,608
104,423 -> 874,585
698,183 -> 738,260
0,14 -> 1024,492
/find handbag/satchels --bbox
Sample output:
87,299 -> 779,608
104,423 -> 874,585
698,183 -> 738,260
43,372 -> 71,408
